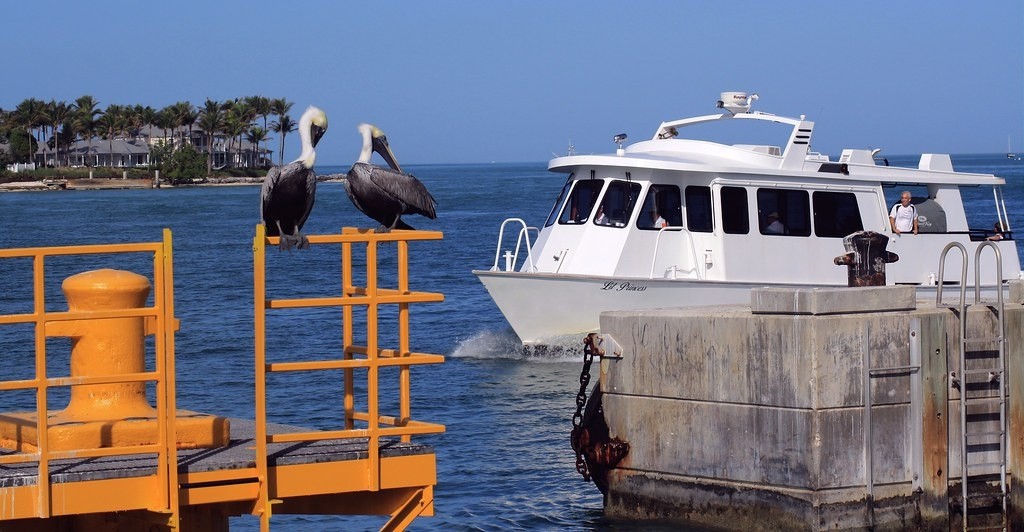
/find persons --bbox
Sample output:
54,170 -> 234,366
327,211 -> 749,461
766,211 -> 789,233
595,205 -> 609,224
647,204 -> 669,228
985,221 -> 1007,241
889,191 -> 918,235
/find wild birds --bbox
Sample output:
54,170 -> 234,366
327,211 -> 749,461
342,123 -> 438,247
260,105 -> 328,252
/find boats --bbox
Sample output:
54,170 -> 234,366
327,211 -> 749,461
470,91 -> 1024,358
